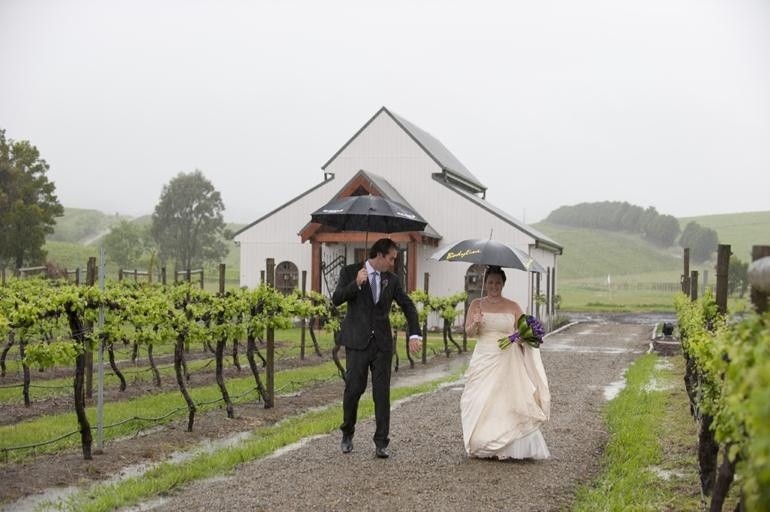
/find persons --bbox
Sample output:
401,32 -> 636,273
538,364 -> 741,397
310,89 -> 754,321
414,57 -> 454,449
333,239 -> 422,457
465,266 -> 550,463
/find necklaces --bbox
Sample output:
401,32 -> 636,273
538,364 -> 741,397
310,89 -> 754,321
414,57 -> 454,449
485,296 -> 504,303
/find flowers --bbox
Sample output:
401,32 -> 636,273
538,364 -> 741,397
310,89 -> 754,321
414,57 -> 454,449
497,312 -> 547,358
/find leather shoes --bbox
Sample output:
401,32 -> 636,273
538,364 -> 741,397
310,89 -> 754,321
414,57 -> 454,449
375,444 -> 390,460
339,435 -> 354,454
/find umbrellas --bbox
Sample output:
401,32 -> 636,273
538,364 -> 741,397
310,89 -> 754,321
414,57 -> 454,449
423,228 -> 548,327
311,183 -> 430,282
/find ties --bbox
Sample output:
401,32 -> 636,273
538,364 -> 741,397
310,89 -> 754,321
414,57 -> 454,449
370,270 -> 378,304
